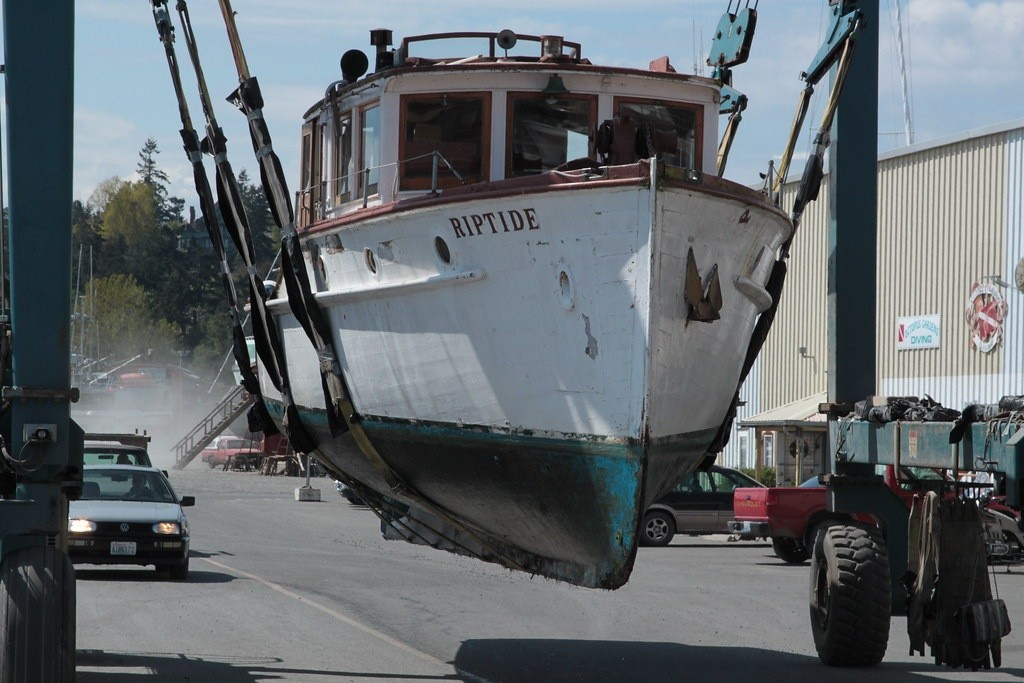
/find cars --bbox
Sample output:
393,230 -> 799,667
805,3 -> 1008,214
637,462 -> 769,548
65,463 -> 195,580
723,469 -> 1024,568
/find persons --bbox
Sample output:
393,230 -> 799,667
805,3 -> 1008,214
125,474 -> 151,497
117,454 -> 129,464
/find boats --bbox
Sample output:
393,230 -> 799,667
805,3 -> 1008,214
231,28 -> 796,589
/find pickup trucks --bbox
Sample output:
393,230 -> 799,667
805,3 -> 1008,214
201,436 -> 264,470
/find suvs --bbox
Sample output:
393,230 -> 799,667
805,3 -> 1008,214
82,428 -> 169,496
